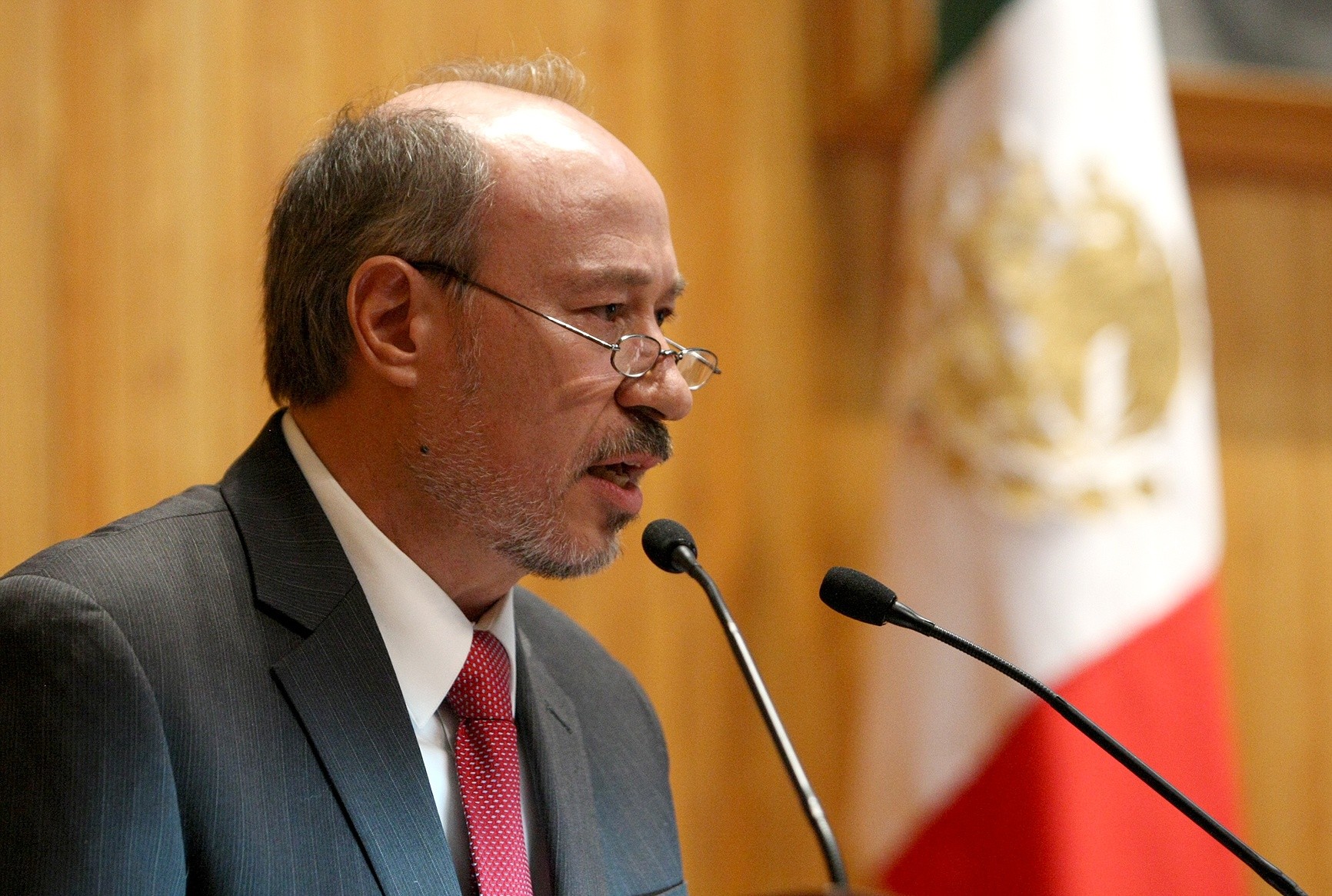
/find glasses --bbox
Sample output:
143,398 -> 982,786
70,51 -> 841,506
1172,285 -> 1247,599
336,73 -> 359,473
405,260 -> 722,391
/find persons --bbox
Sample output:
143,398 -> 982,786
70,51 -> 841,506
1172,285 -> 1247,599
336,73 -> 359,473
0,79 -> 723,896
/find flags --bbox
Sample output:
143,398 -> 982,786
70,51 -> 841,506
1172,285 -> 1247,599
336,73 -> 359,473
859,0 -> 1257,896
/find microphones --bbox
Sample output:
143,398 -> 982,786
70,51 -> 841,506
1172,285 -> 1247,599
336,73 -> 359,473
819,565 -> 1311,896
642,520 -> 851,896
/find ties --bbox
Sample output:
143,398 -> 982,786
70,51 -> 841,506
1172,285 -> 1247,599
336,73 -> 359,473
442,629 -> 535,896
